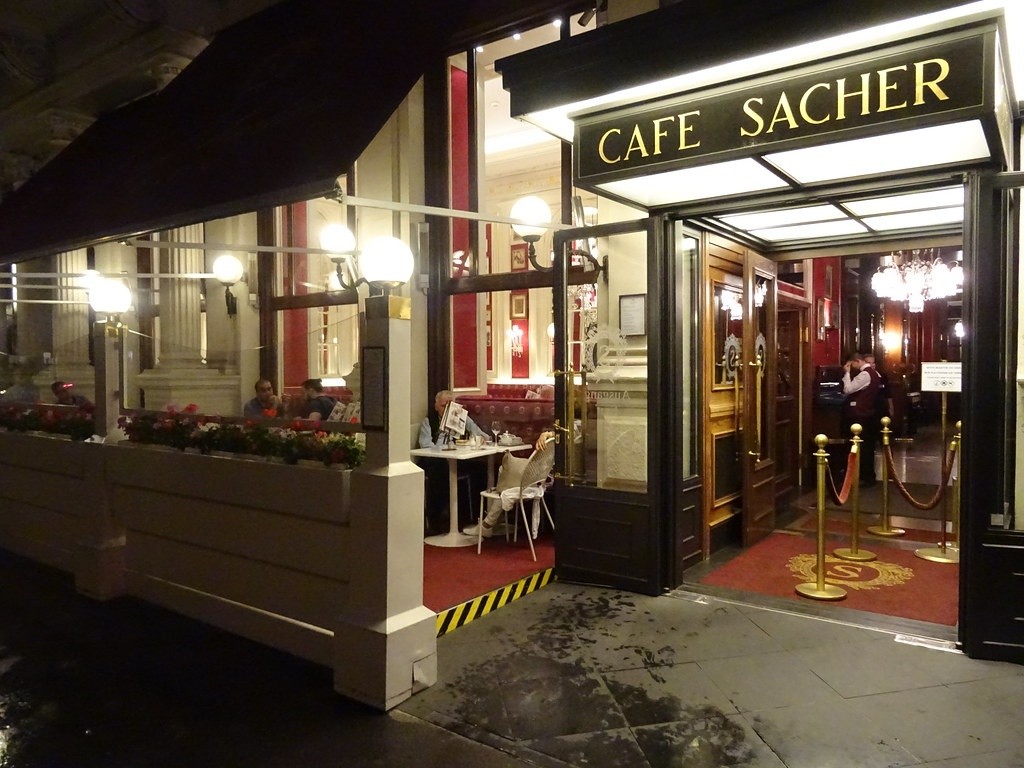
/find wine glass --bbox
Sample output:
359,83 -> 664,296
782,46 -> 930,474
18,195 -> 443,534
491,421 -> 502,448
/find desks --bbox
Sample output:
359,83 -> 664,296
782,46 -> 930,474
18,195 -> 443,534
467,443 -> 533,536
409,442 -> 498,548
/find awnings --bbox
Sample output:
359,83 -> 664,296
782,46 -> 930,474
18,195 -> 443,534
2,1 -> 485,270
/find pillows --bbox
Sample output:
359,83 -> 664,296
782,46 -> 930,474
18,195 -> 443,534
496,449 -> 537,495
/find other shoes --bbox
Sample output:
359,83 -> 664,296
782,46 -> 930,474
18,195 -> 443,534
463,521 -> 493,538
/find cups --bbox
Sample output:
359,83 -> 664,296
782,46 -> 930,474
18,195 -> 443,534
472,436 -> 484,447
512,437 -> 523,443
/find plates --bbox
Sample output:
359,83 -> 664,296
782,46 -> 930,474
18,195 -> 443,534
498,442 -> 524,447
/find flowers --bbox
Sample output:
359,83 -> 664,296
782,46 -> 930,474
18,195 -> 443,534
0,394 -> 366,469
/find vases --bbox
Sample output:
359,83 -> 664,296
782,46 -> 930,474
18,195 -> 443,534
248,455 -> 267,461
210,450 -> 225,456
269,455 -> 288,464
118,439 -> 139,447
224,452 -> 240,458
239,453 -> 251,458
185,447 -> 202,454
330,462 -> 350,469
296,458 -> 324,467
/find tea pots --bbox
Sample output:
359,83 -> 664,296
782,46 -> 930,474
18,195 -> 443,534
498,430 -> 516,442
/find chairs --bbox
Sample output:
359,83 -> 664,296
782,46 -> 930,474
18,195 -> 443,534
477,437 -> 555,561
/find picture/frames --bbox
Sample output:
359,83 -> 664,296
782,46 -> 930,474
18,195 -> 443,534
509,292 -> 528,320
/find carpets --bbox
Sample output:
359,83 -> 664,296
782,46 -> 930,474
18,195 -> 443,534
698,530 -> 961,629
828,480 -> 953,523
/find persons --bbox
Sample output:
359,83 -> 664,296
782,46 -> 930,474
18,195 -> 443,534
1,366 -> 40,403
293,378 -> 334,421
418,389 -> 492,530
243,378 -> 281,421
51,381 -> 90,407
861,353 -> 895,444
464,397 -> 582,537
841,351 -> 878,488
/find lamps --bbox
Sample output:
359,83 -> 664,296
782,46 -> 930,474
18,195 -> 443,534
869,248 -> 963,313
215,255 -> 245,317
359,234 -> 416,295
82,270 -> 132,324
511,195 -> 555,274
318,221 -> 382,295
